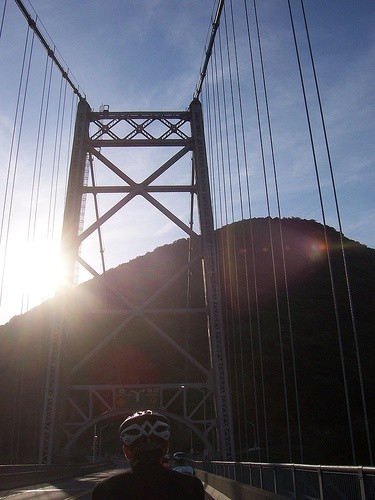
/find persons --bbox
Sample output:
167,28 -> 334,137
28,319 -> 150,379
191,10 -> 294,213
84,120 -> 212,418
92,409 -> 205,500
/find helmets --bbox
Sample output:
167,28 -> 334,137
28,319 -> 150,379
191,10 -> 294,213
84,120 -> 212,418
118,410 -> 171,446
174,452 -> 187,460
